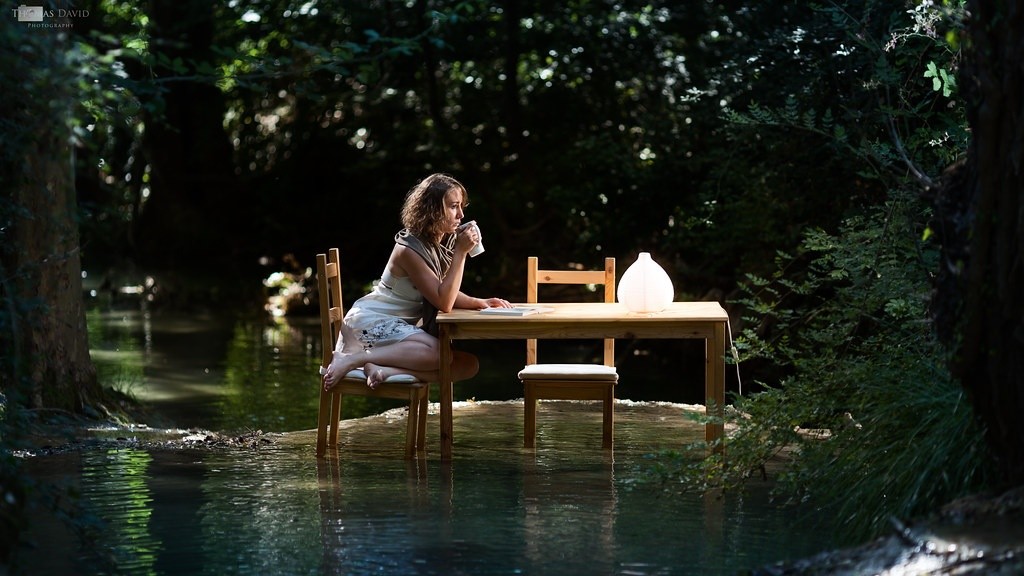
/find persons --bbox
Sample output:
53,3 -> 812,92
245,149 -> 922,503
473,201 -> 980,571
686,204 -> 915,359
324,174 -> 515,394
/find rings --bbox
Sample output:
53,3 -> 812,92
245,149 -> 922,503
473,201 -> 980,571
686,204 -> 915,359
475,240 -> 478,243
501,299 -> 503,301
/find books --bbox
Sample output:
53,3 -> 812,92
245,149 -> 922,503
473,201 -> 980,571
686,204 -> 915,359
479,300 -> 555,316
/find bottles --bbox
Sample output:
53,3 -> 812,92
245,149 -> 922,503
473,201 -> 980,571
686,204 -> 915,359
616,253 -> 674,313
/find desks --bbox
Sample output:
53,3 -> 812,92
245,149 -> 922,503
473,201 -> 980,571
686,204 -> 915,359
435,300 -> 729,463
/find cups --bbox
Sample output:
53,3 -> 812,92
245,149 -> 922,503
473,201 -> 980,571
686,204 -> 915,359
455,220 -> 485,258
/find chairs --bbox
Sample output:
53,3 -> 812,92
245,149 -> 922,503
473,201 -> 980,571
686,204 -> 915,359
517,257 -> 619,450
316,248 -> 429,458
